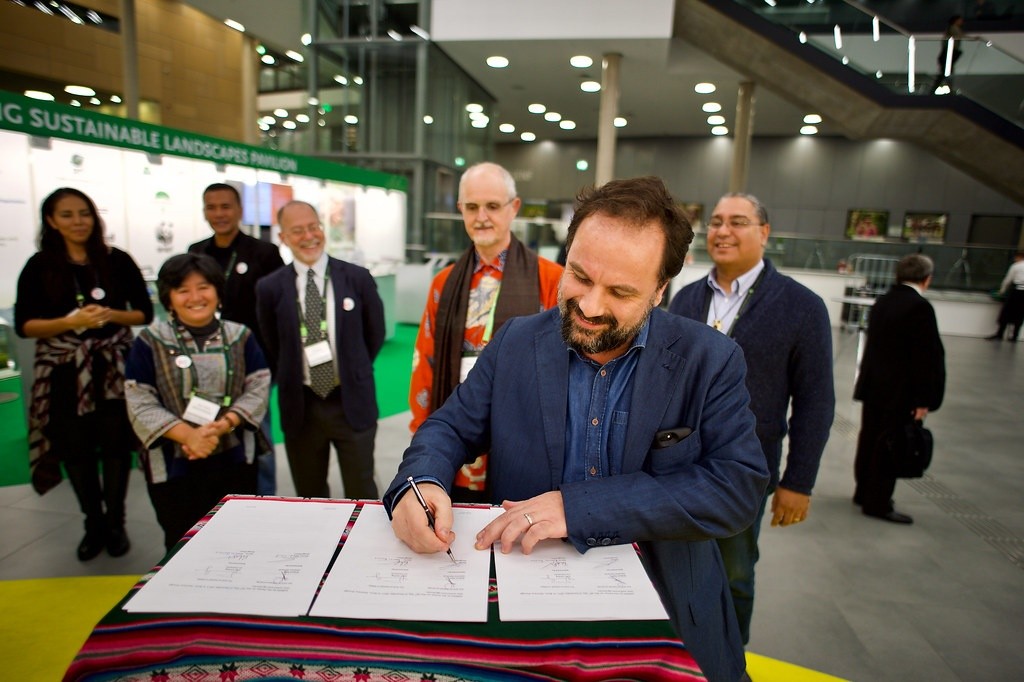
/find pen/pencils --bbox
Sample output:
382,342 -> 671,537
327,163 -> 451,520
407,476 -> 458,567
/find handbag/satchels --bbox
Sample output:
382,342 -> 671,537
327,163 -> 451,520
895,418 -> 933,480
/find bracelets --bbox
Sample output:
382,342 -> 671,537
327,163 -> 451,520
221,416 -> 235,433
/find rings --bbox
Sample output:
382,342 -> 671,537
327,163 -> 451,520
794,518 -> 800,521
524,513 -> 533,526
98,321 -> 102,327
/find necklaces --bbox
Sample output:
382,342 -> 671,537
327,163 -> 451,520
713,294 -> 743,331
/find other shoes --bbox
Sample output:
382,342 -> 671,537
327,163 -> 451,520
853,489 -> 866,505
102,519 -> 130,558
76,519 -> 103,562
984,334 -> 1002,341
1006,337 -> 1018,343
861,509 -> 913,525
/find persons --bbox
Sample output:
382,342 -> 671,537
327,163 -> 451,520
410,161 -> 564,504
382,176 -> 770,682
124,253 -> 271,554
667,191 -> 835,646
256,200 -> 386,499
13,187 -> 155,562
851,255 -> 947,525
937,14 -> 966,75
187,183 -> 286,496
987,253 -> 1024,342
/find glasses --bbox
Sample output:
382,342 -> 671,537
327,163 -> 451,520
458,197 -> 514,213
280,221 -> 325,239
704,214 -> 762,232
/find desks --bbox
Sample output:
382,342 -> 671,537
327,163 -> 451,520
767,233 -> 1024,287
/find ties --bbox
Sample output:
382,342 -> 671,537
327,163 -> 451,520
304,270 -> 336,400
